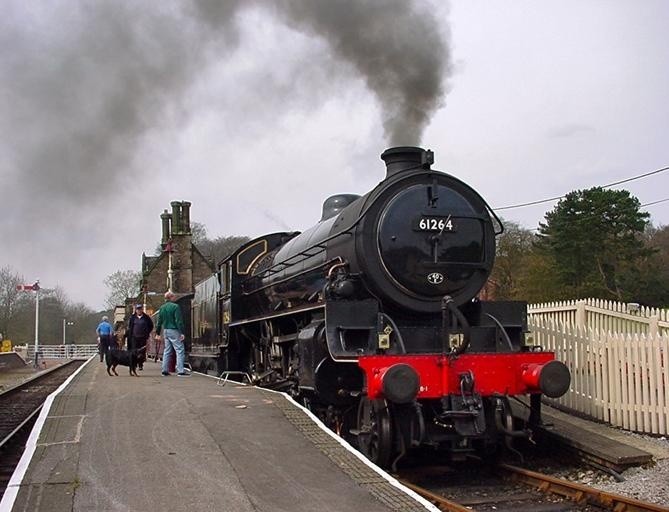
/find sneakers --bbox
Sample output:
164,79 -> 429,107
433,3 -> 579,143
161,370 -> 171,377
176,371 -> 191,377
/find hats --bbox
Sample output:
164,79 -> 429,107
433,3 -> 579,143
135,304 -> 143,309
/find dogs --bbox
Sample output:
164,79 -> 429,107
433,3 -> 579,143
106,350 -> 146,377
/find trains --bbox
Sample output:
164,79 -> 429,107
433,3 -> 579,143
146,147 -> 570,472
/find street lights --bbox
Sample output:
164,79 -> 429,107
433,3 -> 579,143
34,278 -> 40,351
63,319 -> 74,344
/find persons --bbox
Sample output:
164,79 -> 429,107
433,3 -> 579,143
97,329 -> 129,351
154,291 -> 191,377
168,349 -> 177,375
129,304 -> 153,371
96,315 -> 113,363
